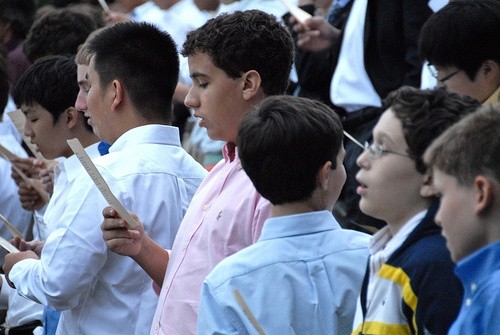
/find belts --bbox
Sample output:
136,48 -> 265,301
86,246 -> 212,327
341,107 -> 376,122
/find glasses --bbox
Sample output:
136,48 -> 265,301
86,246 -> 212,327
363,140 -> 416,160
427,63 -> 461,81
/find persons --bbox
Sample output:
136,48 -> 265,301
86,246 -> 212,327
0,0 -> 500,335
2,22 -> 211,335
